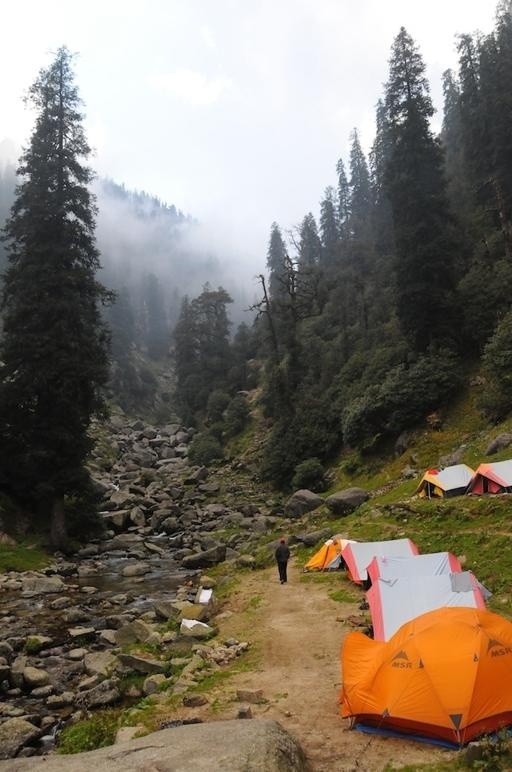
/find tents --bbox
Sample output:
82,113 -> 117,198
328,538 -> 418,585
338,605 -> 512,751
364,551 -> 462,590
463,458 -> 511,496
362,570 -> 487,642
416,462 -> 474,498
303,538 -> 360,572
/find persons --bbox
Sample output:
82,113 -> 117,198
275,538 -> 289,584
